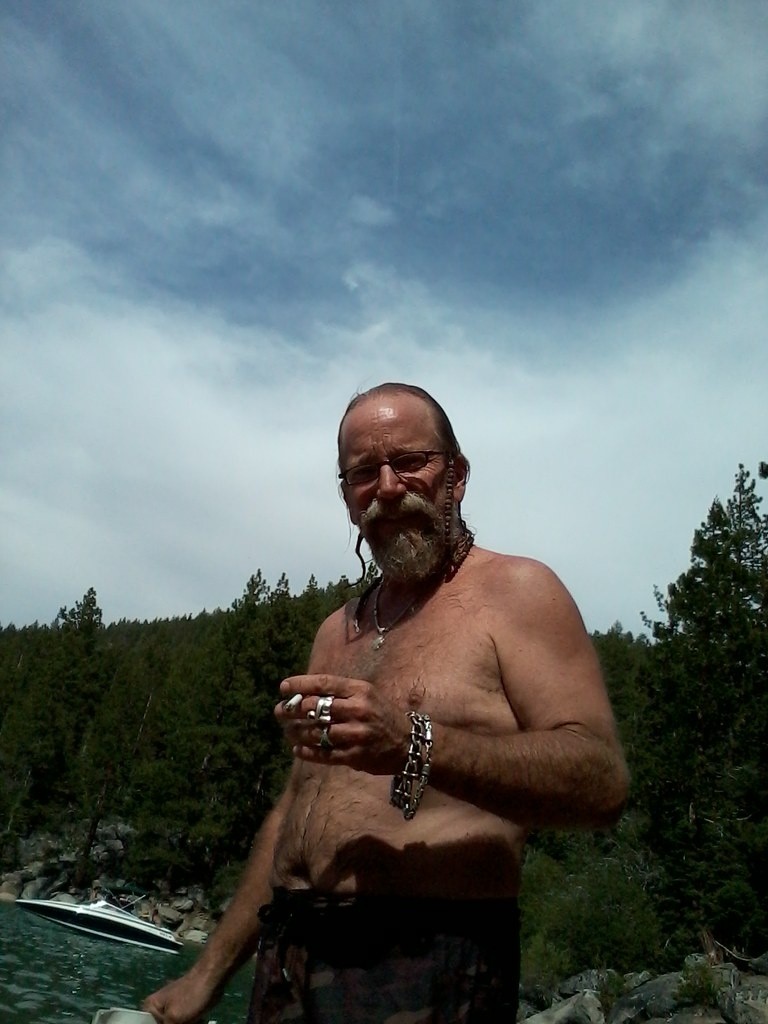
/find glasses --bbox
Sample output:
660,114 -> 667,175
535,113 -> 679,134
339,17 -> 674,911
338,451 -> 452,487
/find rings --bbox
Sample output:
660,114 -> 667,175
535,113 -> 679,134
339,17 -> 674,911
320,725 -> 333,748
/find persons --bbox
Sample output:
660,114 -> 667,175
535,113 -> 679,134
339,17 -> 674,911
90,887 -> 104,901
141,375 -> 633,1024
152,909 -> 161,925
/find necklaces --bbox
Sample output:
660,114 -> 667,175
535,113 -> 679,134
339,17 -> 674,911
371,578 -> 420,650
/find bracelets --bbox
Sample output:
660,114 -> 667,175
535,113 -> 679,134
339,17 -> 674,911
388,711 -> 434,820
307,694 -> 335,723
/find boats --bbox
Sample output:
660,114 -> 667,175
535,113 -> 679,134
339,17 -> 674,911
15,888 -> 184,955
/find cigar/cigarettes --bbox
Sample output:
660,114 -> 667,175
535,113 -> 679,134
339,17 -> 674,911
284,693 -> 307,713
